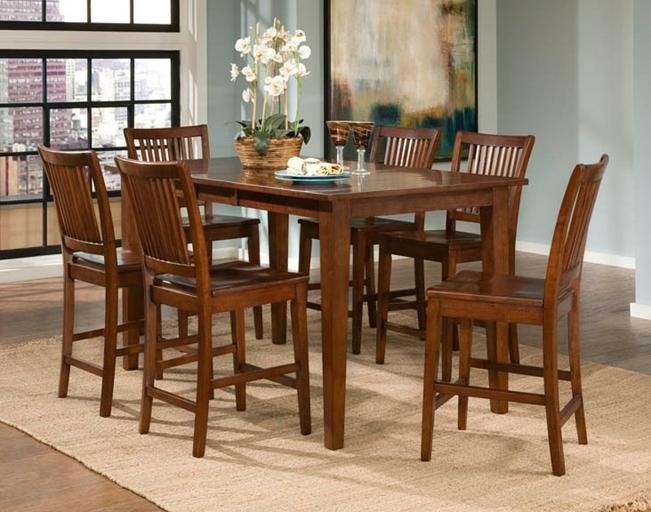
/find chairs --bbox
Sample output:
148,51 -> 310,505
116,124 -> 535,380
421,155 -> 609,476
116,155 -> 310,458
39,145 -> 196,418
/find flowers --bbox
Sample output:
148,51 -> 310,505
228,14 -> 313,154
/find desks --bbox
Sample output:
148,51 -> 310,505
174,156 -> 530,450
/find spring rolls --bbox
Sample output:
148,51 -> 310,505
287,157 -> 343,176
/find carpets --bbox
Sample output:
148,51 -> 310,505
2,299 -> 650,512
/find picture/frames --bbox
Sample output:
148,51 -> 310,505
322,0 -> 479,163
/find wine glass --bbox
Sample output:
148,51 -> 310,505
327,120 -> 375,175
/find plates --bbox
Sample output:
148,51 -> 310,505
275,169 -> 352,185
